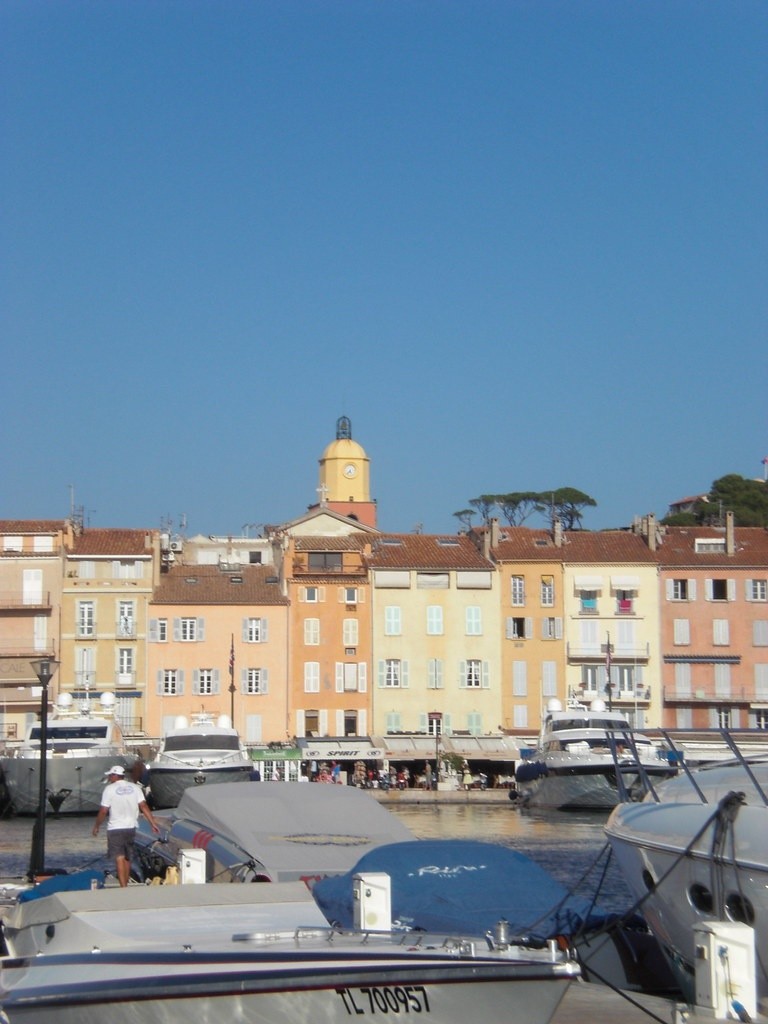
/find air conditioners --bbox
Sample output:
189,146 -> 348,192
170,541 -> 182,552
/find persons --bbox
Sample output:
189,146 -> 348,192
93,765 -> 161,888
301,758 -> 488,792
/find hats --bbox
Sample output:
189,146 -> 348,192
105,766 -> 125,775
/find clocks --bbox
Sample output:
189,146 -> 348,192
342,461 -> 359,479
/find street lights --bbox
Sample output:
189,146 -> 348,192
27,658 -> 61,885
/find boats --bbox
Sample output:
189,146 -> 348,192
146,705 -> 260,810
1,672 -> 142,818
507,628 -> 684,811
602,725 -> 768,1017
